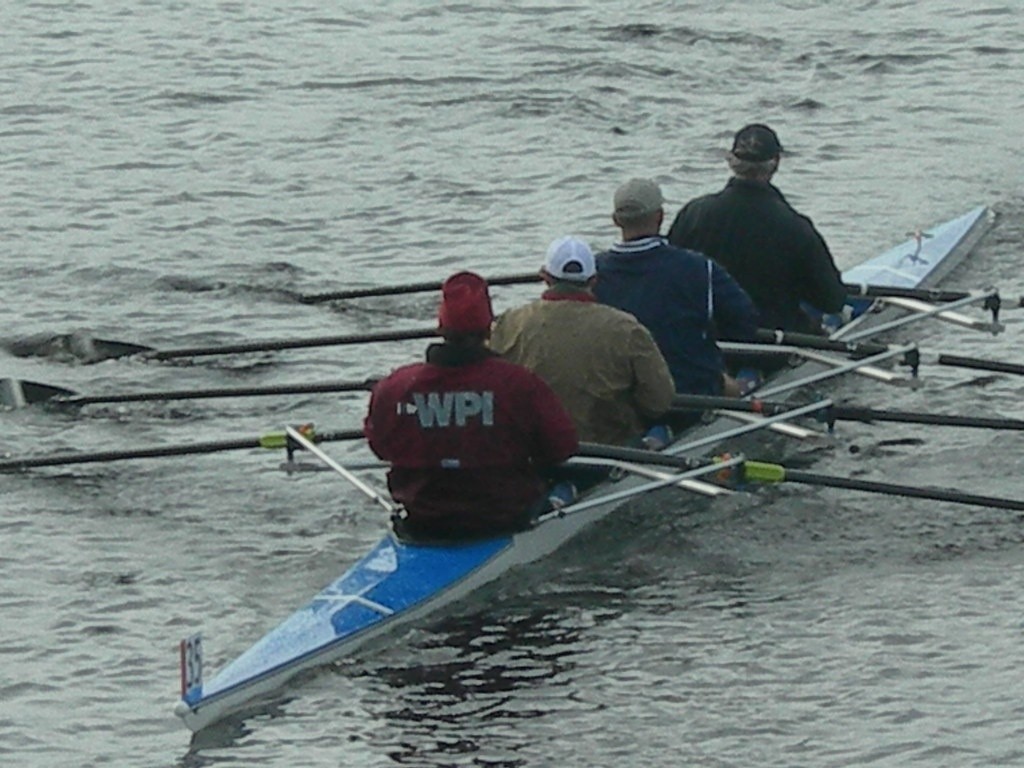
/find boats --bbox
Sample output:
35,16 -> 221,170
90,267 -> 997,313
169,202 -> 1003,732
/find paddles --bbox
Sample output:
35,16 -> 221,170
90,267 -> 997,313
0,376 -> 384,410
573,440 -> 1024,511
748,324 -> 1024,377
1,423 -> 365,474
674,389 -> 1024,438
41,327 -> 447,367
843,280 -> 1023,308
296,269 -> 546,304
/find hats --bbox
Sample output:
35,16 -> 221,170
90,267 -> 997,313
732,124 -> 784,160
543,235 -> 596,280
439,272 -> 493,333
614,177 -> 667,217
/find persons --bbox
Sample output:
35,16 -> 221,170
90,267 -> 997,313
362,270 -> 579,535
668,123 -> 849,370
488,236 -> 676,447
590,175 -> 754,432
